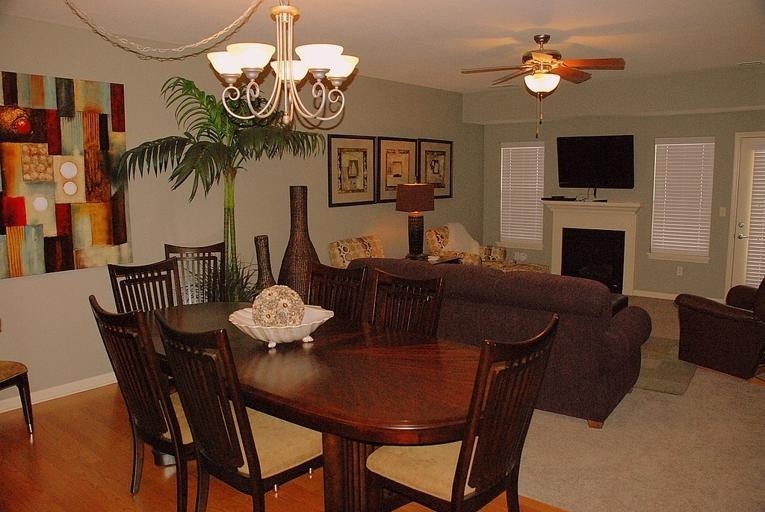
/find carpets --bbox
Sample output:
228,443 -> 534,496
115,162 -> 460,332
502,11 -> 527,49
632,335 -> 696,395
513,298 -> 764,512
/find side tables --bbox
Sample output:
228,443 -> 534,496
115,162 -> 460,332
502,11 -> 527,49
424,252 -> 462,264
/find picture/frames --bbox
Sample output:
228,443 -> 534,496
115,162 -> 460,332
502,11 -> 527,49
377,136 -> 417,202
417,138 -> 452,200
327,134 -> 377,207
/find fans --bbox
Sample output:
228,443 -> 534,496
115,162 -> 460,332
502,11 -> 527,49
460,34 -> 625,138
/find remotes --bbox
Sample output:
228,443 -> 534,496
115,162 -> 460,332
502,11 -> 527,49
431,255 -> 459,265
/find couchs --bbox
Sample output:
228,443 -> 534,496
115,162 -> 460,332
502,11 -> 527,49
345,257 -> 651,428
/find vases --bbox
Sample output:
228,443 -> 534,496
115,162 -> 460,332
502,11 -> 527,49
278,186 -> 322,305
251,234 -> 278,303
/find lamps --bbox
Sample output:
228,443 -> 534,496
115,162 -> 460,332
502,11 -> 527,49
395,183 -> 434,261
524,69 -> 560,139
61,0 -> 360,128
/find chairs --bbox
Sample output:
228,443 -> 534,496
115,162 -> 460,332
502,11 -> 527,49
328,236 -> 384,269
674,275 -> 765,379
426,224 -> 550,274
0,360 -> 33,438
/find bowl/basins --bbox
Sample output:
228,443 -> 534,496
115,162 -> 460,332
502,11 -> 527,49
227,308 -> 336,348
237,350 -> 330,386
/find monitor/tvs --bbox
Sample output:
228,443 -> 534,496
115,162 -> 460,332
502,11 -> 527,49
556,135 -> 634,190
